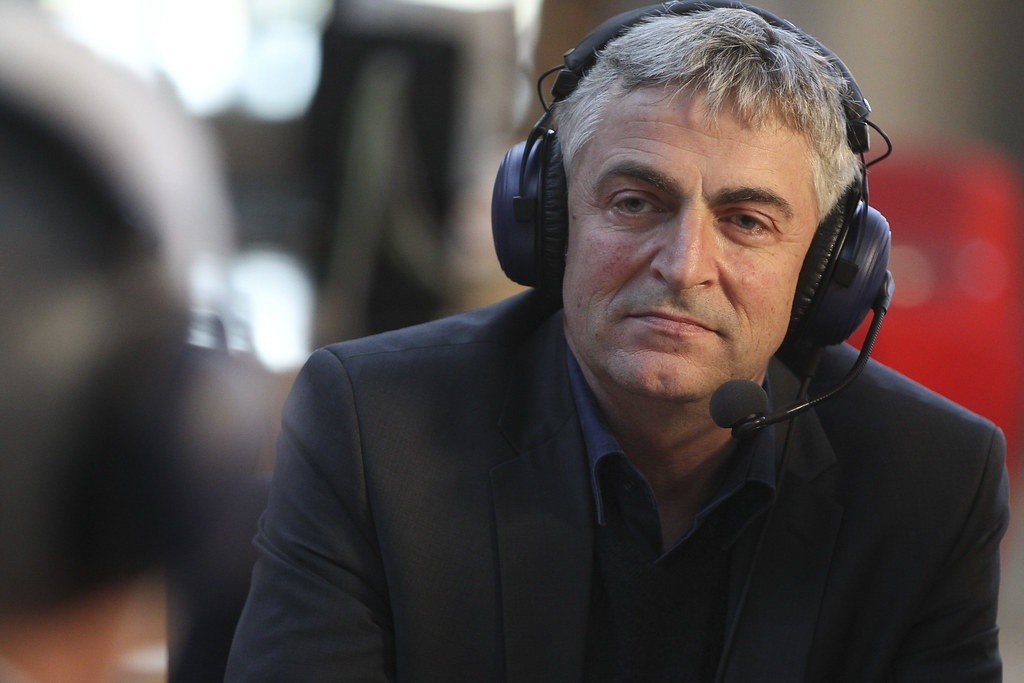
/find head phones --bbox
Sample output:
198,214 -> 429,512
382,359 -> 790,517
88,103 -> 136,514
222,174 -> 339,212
490,0 -> 894,340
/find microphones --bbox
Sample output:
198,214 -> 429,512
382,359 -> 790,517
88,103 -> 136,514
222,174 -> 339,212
711,308 -> 887,439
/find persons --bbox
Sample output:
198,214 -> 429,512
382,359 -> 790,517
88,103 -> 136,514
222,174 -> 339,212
219,0 -> 1011,683
